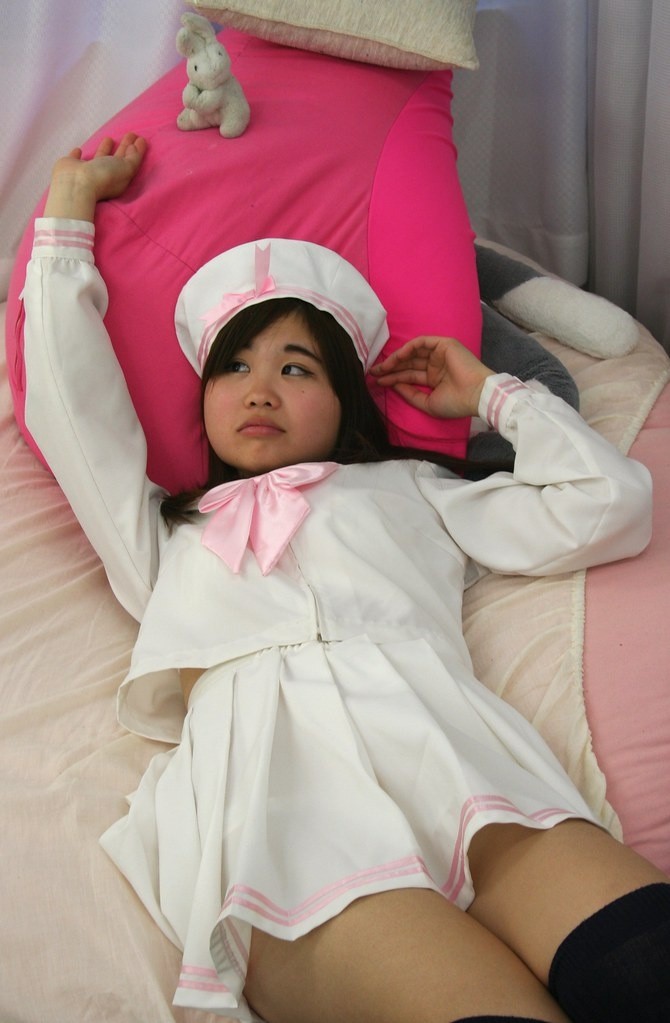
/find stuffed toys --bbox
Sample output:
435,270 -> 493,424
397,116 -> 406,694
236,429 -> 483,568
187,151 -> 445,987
468,243 -> 640,471
176,13 -> 250,138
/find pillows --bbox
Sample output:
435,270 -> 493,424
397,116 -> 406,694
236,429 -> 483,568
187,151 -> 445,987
194,0 -> 483,71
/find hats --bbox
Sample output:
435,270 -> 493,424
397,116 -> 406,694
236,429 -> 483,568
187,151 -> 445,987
173,237 -> 389,378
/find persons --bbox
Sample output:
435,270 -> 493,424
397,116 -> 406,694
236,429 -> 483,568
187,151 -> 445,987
19,132 -> 670,1022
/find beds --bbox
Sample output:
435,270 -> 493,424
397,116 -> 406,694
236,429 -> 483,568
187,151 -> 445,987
0,298 -> 669,1023
